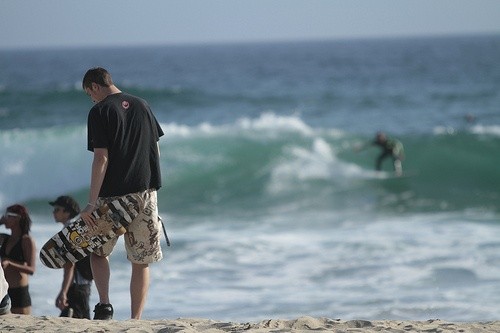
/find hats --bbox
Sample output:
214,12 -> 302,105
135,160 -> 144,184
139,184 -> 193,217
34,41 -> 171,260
48,195 -> 81,213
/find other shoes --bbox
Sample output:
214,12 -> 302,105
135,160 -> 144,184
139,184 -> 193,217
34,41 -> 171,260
93,302 -> 113,322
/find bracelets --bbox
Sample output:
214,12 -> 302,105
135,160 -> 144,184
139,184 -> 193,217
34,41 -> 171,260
87,201 -> 97,206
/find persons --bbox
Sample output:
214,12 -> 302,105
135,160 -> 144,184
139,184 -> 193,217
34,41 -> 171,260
362,133 -> 404,173
79,66 -> 172,319
49,193 -> 94,318
1,202 -> 37,313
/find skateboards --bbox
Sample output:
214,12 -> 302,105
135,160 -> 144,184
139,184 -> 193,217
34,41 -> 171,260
39,193 -> 144,269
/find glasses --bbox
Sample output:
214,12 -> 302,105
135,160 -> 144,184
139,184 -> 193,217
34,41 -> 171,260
5,212 -> 20,219
